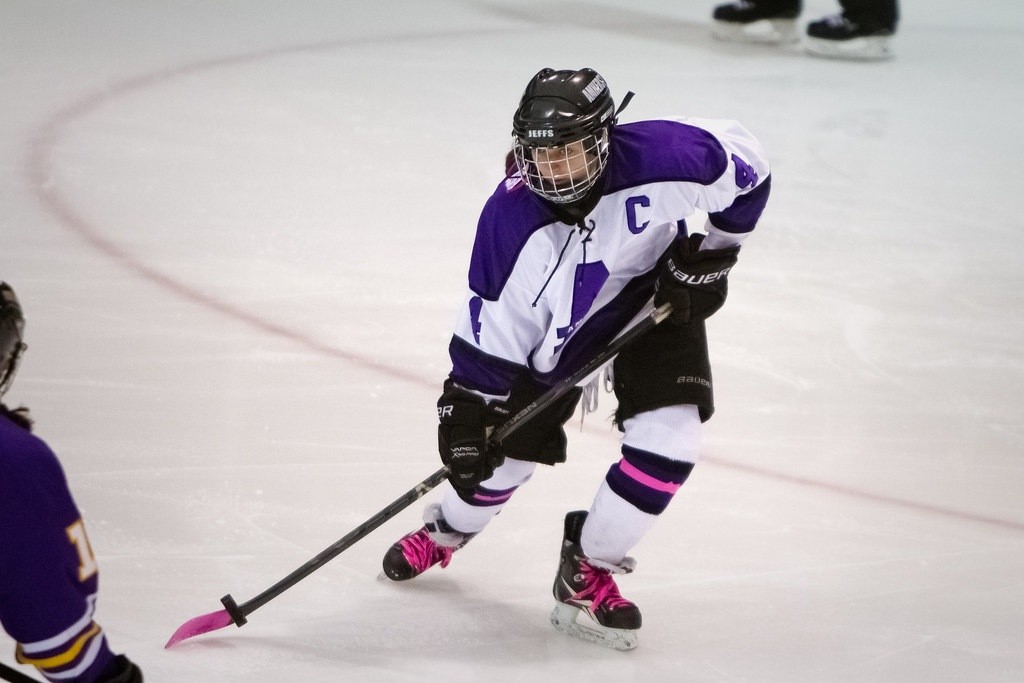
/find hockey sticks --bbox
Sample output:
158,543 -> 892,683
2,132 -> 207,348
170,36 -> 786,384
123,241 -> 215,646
165,302 -> 675,650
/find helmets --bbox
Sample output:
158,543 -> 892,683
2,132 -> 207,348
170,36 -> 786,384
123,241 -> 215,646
511,68 -> 635,203
0,280 -> 27,397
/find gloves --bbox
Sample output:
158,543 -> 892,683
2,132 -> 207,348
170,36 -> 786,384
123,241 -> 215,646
438,378 -> 512,498
653,233 -> 741,325
89,654 -> 142,683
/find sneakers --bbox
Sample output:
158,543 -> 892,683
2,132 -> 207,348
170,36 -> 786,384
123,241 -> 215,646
378,499 -> 481,581
711,0 -> 804,42
803,14 -> 897,59
549,510 -> 642,650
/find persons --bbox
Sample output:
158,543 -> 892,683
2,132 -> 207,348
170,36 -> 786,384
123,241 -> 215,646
383,67 -> 772,630
0,280 -> 142,683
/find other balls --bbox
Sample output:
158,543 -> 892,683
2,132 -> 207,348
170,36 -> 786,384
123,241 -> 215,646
219,594 -> 248,629
